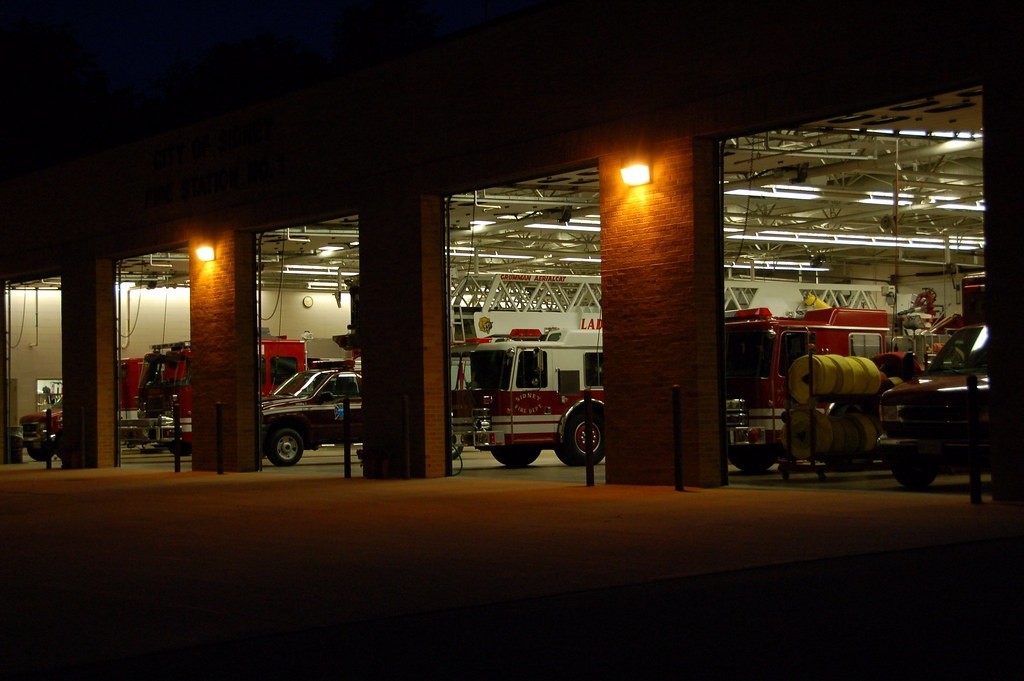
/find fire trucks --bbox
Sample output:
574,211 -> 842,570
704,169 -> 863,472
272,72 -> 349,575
451,325 -> 605,467
722,302 -> 964,475
19,330 -> 361,462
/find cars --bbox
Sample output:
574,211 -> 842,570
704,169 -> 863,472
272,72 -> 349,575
875,320 -> 990,489
262,358 -> 363,467
